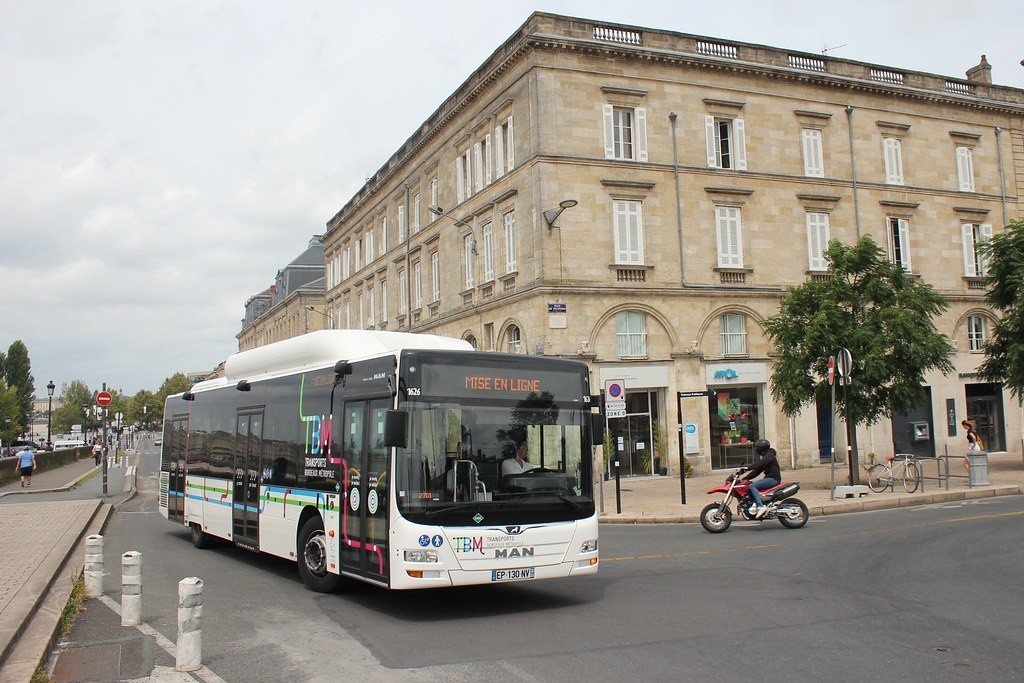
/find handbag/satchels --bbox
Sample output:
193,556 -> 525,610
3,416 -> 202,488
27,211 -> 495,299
92,447 -> 96,458
977,441 -> 984,451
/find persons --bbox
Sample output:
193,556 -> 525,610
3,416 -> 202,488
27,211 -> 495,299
91,438 -> 103,468
962,420 -> 980,474
739,438 -> 781,519
16,447 -> 36,487
502,442 -> 541,477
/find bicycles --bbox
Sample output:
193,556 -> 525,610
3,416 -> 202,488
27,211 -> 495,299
868,453 -> 920,494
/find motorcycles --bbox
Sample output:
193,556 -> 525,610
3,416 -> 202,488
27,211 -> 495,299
700,459 -> 810,534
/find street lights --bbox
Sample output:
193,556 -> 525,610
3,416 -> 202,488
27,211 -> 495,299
4,416 -> 12,452
45,380 -> 56,451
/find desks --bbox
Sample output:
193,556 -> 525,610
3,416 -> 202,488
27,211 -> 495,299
718,442 -> 755,468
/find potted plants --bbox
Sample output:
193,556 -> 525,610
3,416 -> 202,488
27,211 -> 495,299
653,418 -> 668,475
603,427 -> 614,481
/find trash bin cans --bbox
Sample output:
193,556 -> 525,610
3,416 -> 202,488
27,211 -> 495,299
967,453 -> 990,487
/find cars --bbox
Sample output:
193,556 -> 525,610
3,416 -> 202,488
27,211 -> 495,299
0,424 -> 145,458
154,437 -> 162,446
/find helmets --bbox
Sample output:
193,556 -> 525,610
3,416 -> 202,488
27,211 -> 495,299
755,439 -> 770,453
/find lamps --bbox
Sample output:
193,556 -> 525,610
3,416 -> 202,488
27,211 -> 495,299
541,198 -> 578,226
305,305 -> 335,329
427,205 -> 478,256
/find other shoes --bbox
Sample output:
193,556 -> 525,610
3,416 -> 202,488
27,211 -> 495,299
755,505 -> 767,518
22,481 -> 24,487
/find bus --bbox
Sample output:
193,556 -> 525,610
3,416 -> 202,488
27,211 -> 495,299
157,329 -> 607,595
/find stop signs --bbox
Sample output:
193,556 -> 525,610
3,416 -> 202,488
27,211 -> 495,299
96,391 -> 113,407
828,356 -> 835,385
